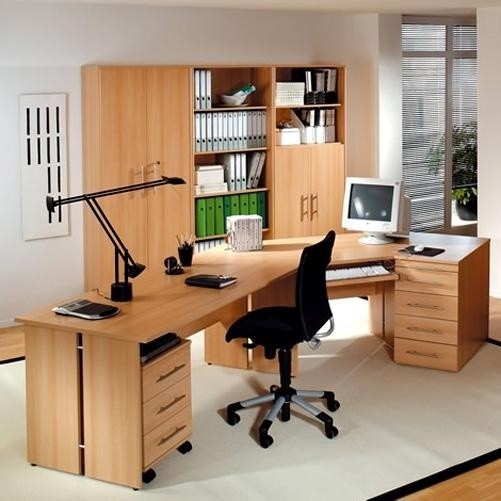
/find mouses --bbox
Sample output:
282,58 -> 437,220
413,246 -> 425,253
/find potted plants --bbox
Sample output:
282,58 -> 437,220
425,122 -> 478,220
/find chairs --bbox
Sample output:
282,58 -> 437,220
221,231 -> 343,449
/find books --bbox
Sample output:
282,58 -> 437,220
226,213 -> 263,252
185,272 -> 239,290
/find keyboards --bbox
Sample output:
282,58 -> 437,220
326,265 -> 390,281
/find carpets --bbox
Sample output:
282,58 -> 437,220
0,298 -> 501,501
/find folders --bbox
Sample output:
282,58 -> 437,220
195,69 -> 211,110
194,110 -> 266,237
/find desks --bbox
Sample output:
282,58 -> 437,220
18,232 -> 492,494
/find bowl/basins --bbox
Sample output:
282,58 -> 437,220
220,95 -> 246,106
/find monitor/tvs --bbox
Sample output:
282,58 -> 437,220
341,177 -> 412,245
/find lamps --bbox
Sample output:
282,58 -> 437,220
47,176 -> 188,305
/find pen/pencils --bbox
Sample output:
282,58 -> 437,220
175,232 -> 196,248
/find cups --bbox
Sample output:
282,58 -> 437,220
178,245 -> 194,266
